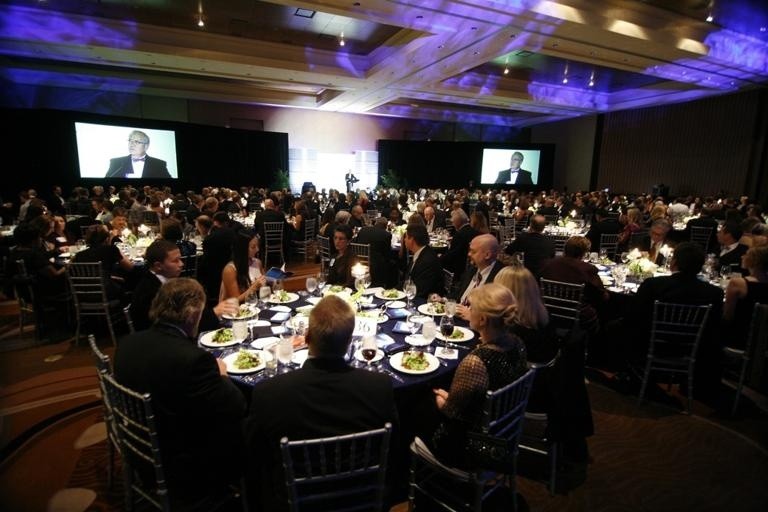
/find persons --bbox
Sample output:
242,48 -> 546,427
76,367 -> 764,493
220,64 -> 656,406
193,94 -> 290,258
104,130 -> 173,180
0,178 -> 768,392
114,277 -> 247,511
346,170 -> 357,191
247,295 -> 410,512
494,151 -> 535,187
417,282 -> 529,480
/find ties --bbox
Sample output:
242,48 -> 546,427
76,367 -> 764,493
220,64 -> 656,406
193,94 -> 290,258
462,273 -> 482,309
650,243 -> 656,263
428,220 -> 431,225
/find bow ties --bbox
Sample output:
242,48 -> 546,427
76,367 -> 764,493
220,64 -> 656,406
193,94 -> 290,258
512,171 -> 519,174
133,158 -> 146,162
724,246 -> 730,250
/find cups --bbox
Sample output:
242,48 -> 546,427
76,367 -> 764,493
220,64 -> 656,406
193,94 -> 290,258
719,265 -> 730,280
74,239 -> 145,257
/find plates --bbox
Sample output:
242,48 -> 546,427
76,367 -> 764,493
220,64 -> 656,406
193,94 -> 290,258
200,267 -> 476,373
590,262 -> 641,293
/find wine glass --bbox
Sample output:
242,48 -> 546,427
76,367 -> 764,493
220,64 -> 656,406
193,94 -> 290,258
595,247 -> 630,264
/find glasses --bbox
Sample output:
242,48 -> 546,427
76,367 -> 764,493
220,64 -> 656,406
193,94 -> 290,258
512,159 -> 520,161
130,139 -> 146,145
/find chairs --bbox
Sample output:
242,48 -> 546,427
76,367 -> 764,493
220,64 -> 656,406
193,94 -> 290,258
8,259 -> 57,343
633,299 -> 713,417
406,365 -> 537,512
538,276 -> 611,373
516,347 -> 567,498
86,333 -> 118,490
718,302 -> 767,421
278,422 -> 393,512
94,367 -> 252,512
62,260 -> 120,347
122,303 -> 137,335
